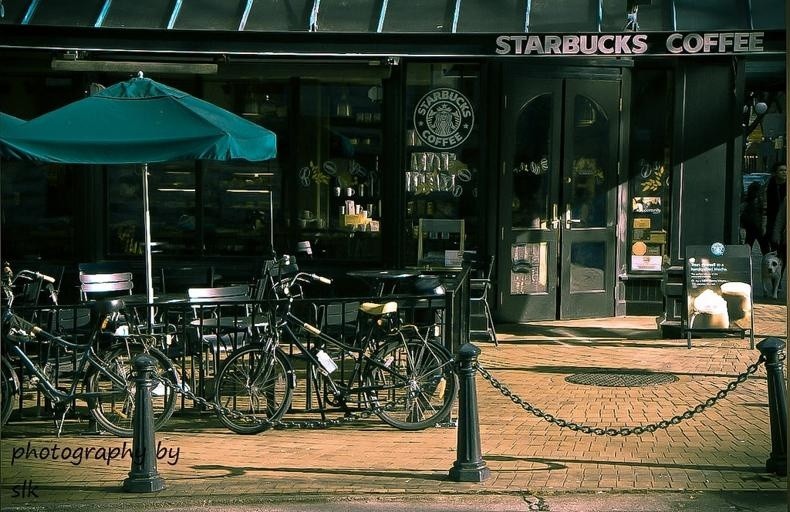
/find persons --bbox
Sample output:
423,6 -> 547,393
741,179 -> 769,258
746,162 -> 787,292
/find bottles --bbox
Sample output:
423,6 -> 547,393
316,349 -> 339,375
333,183 -> 370,232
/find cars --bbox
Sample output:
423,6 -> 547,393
742,175 -> 765,196
750,171 -> 774,185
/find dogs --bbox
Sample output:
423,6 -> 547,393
762,252 -> 782,300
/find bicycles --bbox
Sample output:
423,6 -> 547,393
207,251 -> 457,435
0,260 -> 181,436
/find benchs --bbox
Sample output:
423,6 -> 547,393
0,257 -> 397,418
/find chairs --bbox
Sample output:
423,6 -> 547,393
469,255 -> 501,347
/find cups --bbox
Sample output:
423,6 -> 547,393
720,282 -> 750,323
689,302 -> 730,334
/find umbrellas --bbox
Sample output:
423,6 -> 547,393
0,67 -> 280,332
